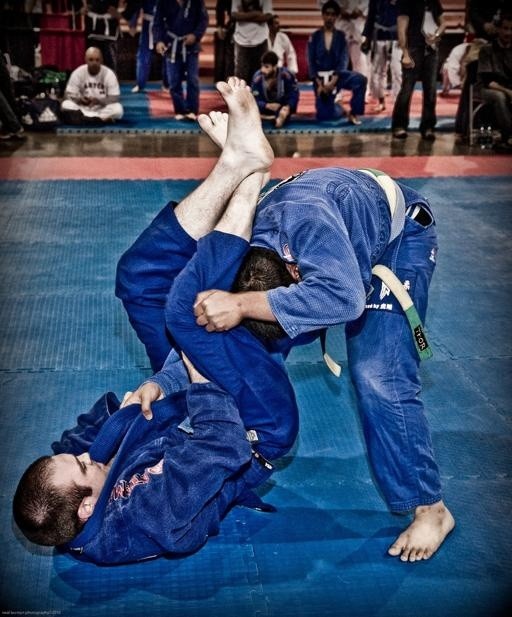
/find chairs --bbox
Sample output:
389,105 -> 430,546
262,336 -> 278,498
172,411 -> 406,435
464,60 -> 488,147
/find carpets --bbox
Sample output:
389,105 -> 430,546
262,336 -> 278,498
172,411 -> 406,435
2,151 -> 512,616
53,80 -> 459,135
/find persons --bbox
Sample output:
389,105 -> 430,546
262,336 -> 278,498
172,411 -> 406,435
121,165 -> 455,562
1,0 -> 512,144
14,76 -> 299,565
